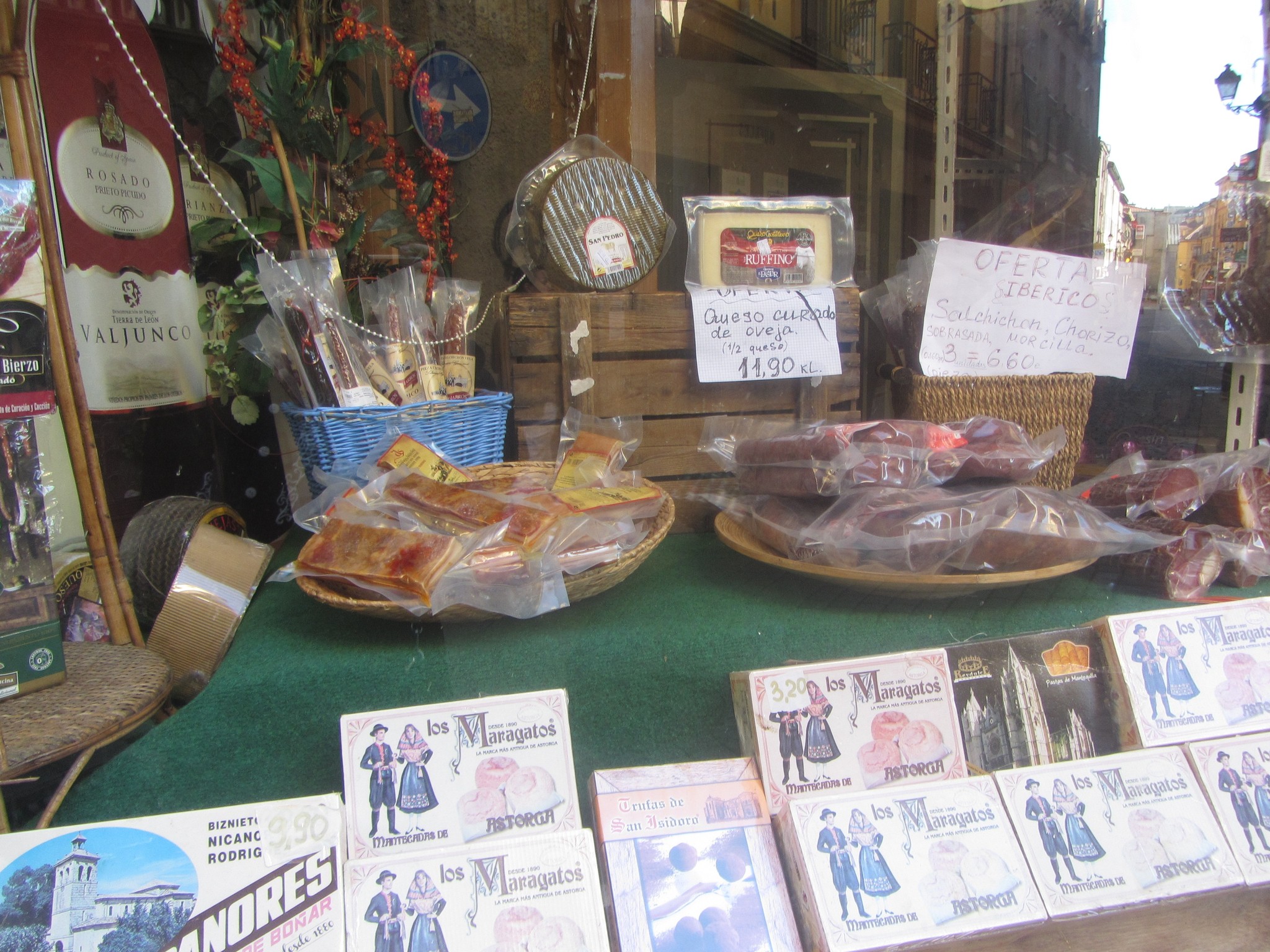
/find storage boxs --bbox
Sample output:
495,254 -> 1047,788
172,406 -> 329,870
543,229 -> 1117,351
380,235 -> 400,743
500,277 -> 865,544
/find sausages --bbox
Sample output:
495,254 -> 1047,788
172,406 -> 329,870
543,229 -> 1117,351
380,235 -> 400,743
273,298 -> 469,415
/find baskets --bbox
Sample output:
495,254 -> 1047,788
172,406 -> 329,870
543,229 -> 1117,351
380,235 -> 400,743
899,365 -> 1096,491
294,461 -> 678,624
279,387 -> 515,498
